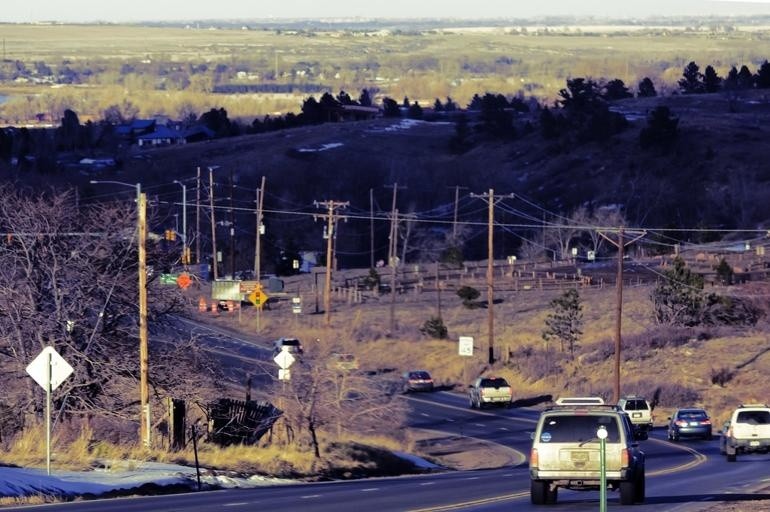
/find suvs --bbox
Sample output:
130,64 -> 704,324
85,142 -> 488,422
719,404 -> 770,461
529,405 -> 643,505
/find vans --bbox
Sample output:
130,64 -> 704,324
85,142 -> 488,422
618,397 -> 652,430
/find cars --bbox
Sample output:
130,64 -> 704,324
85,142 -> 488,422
468,376 -> 513,408
404,370 -> 433,393
275,338 -> 303,361
325,354 -> 358,377
218,270 -> 276,288
668,408 -> 712,441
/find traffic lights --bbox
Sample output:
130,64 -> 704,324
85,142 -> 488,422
166,230 -> 176,241
182,247 -> 190,265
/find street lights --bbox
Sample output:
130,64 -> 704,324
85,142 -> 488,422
173,180 -> 186,271
90,181 -> 141,198
